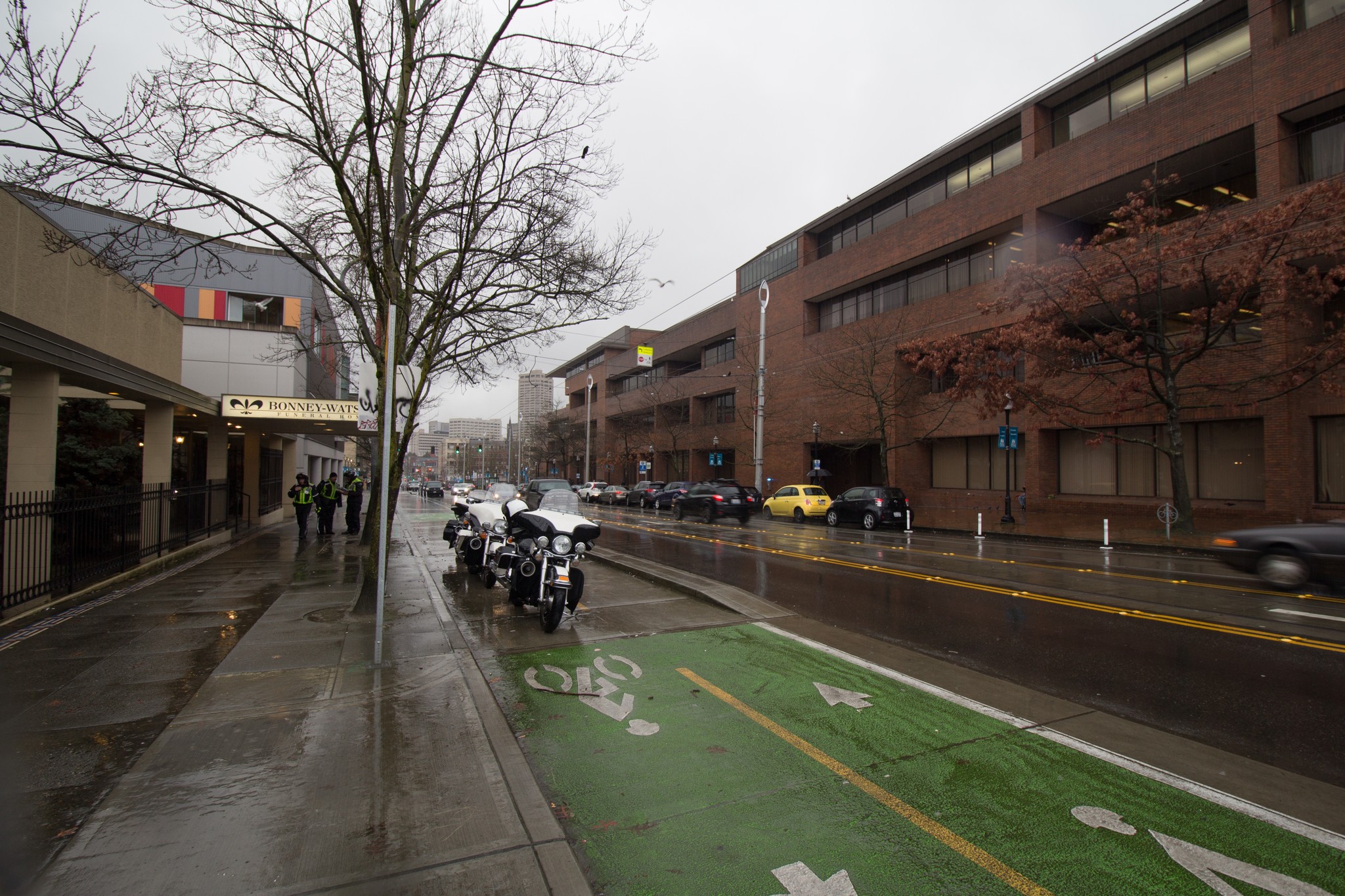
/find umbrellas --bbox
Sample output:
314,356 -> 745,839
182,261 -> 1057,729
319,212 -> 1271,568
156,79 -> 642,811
596,478 -> 605,481
806,468 -> 832,477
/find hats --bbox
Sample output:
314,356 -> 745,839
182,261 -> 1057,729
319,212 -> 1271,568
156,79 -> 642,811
329,472 -> 338,478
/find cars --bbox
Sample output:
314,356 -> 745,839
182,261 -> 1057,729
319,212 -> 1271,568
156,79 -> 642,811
524,479 -> 579,513
1209,517 -> 1345,596
401,480 -> 915,533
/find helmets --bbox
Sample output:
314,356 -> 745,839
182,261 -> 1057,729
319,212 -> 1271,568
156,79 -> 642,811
295,472 -> 309,481
347,470 -> 357,478
504,499 -> 529,524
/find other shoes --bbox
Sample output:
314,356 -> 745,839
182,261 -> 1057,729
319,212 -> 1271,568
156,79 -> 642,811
319,531 -> 325,534
353,530 -> 359,535
326,531 -> 335,534
299,530 -> 308,539
342,529 -> 352,534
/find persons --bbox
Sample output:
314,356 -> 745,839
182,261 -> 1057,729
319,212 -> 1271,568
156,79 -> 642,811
338,469 -> 364,534
315,472 -> 343,535
593,480 -> 595,482
367,480 -> 370,492
1018,476 -> 1026,511
567,478 -> 575,486
610,482 -> 613,485
287,472 -> 320,534
813,476 -> 826,491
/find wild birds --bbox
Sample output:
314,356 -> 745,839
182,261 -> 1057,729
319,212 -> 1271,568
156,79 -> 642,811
582,146 -> 589,159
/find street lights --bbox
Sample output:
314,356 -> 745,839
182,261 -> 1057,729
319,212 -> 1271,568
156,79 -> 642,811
521,464 -> 524,483
487,468 -> 489,478
535,461 -> 538,478
712,436 -> 718,482
490,425 -> 511,483
649,445 -> 654,482
479,469 -> 481,489
999,394 -> 1017,526
477,439 -> 483,490
506,465 -> 508,483
553,459 -> 556,478
576,455 -> 580,485
812,422 -> 820,485
458,474 -> 459,480
454,476 -> 455,481
607,451 -> 612,484
467,472 -> 469,482
450,475 -> 452,481
495,466 -> 498,483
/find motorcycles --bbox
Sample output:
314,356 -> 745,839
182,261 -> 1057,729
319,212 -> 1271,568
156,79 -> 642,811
441,483 -> 531,589
498,489 -> 602,634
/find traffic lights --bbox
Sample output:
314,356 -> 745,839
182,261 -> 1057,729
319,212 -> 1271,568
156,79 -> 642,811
455,444 -> 483,454
412,469 -> 432,473
398,446 -> 400,451
